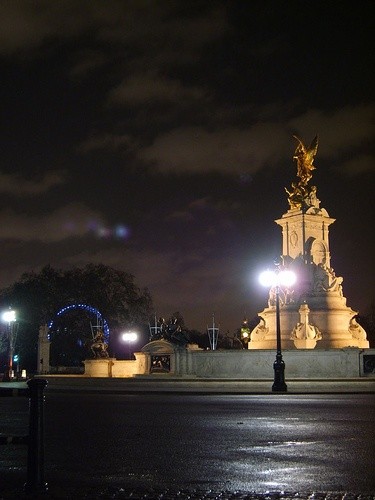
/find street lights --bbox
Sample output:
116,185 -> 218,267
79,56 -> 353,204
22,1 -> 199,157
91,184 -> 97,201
0,304 -> 18,382
120,328 -> 138,360
257,256 -> 297,394
